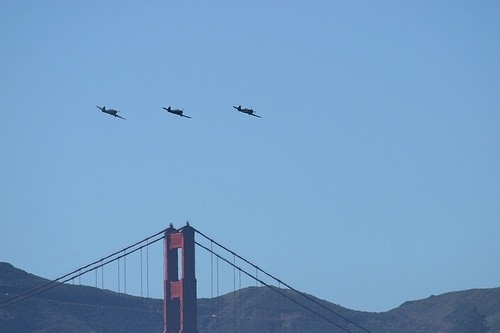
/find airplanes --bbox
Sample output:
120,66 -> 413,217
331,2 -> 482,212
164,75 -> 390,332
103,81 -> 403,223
163,105 -> 190,119
232,105 -> 262,118
95,105 -> 126,120
